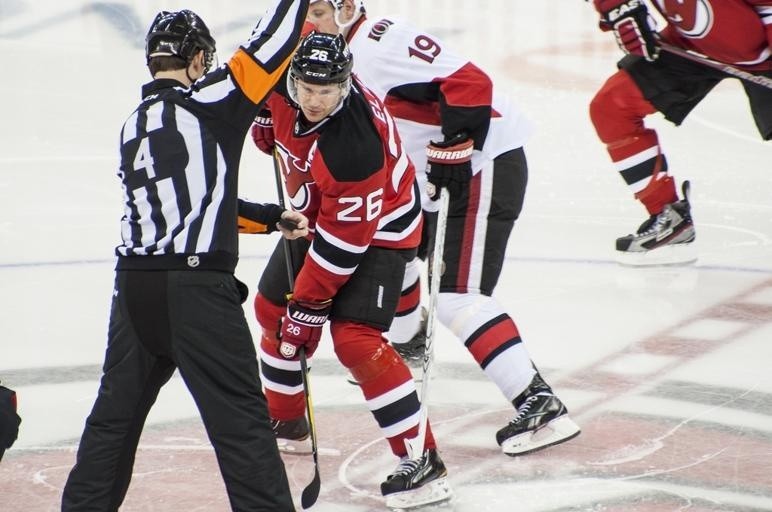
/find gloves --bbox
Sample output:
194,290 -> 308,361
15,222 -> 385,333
426,138 -> 473,206
599,1 -> 660,63
276,291 -> 333,361
251,111 -> 275,155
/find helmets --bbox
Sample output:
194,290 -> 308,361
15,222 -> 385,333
308,0 -> 366,31
290,31 -> 354,83
145,9 -> 218,72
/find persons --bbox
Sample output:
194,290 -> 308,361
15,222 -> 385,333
305,0 -> 571,446
62,0 -> 311,512
251,30 -> 451,500
588,1 -> 772,266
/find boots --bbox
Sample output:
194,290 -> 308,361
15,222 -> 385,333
496,361 -> 568,445
346,306 -> 428,384
615,180 -> 695,252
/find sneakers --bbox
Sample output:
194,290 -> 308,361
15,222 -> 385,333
270,416 -> 310,441
380,448 -> 447,495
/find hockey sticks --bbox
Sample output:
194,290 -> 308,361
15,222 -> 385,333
404,187 -> 451,461
272,143 -> 320,509
